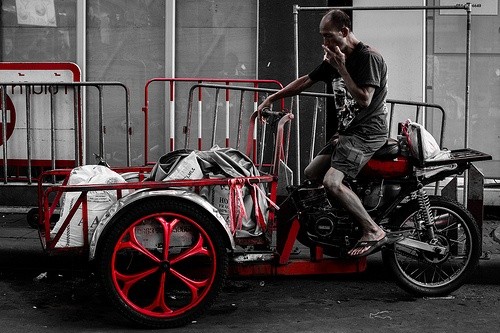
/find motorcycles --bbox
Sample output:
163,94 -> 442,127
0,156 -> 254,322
29,108 -> 493,327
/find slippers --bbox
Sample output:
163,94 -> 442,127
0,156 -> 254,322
350,235 -> 388,259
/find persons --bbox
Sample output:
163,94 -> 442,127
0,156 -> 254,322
255,8 -> 390,261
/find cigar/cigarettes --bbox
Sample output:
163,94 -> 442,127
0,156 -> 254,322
322,51 -> 328,61
